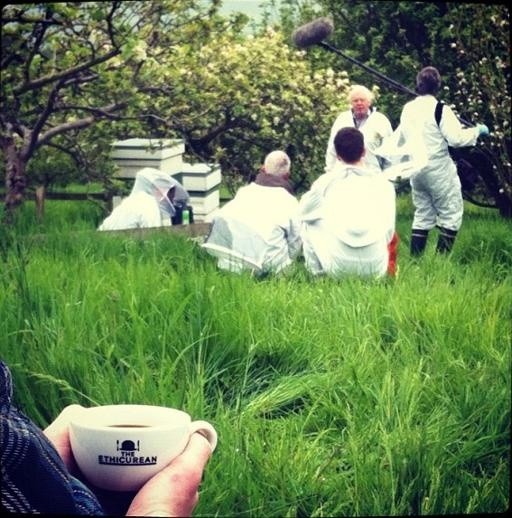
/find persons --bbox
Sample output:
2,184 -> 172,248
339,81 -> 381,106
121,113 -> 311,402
323,84 -> 397,179
298,127 -> 401,282
381,65 -> 489,265
202,149 -> 302,276
0,354 -> 213,518
96,166 -> 192,232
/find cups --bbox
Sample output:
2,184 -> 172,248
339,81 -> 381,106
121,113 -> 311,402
68,404 -> 218,492
